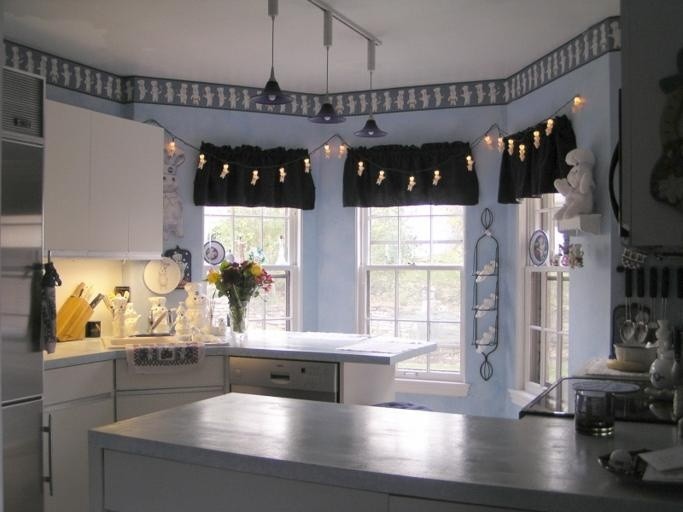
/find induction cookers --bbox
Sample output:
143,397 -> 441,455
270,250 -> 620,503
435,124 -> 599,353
518,377 -> 683,426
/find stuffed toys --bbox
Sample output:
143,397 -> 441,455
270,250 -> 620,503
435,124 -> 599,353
554,149 -> 594,220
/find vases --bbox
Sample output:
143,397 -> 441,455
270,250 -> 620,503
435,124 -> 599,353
226,301 -> 248,343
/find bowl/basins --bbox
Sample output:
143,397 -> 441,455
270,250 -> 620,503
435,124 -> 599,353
613,343 -> 659,371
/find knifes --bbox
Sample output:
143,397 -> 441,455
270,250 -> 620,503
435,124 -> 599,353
73,282 -> 105,310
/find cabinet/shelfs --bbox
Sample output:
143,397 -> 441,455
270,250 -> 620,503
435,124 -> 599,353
116,353 -> 226,420
471,208 -> 499,379
44,361 -> 116,511
46,98 -> 164,259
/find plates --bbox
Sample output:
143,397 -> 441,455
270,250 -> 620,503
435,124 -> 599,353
529,229 -> 548,267
204,241 -> 225,265
144,257 -> 181,294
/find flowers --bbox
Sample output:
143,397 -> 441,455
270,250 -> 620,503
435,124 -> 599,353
209,261 -> 275,301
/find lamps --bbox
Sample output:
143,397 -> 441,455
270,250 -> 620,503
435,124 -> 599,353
250,0 -> 294,105
308,10 -> 346,123
353,36 -> 388,137
